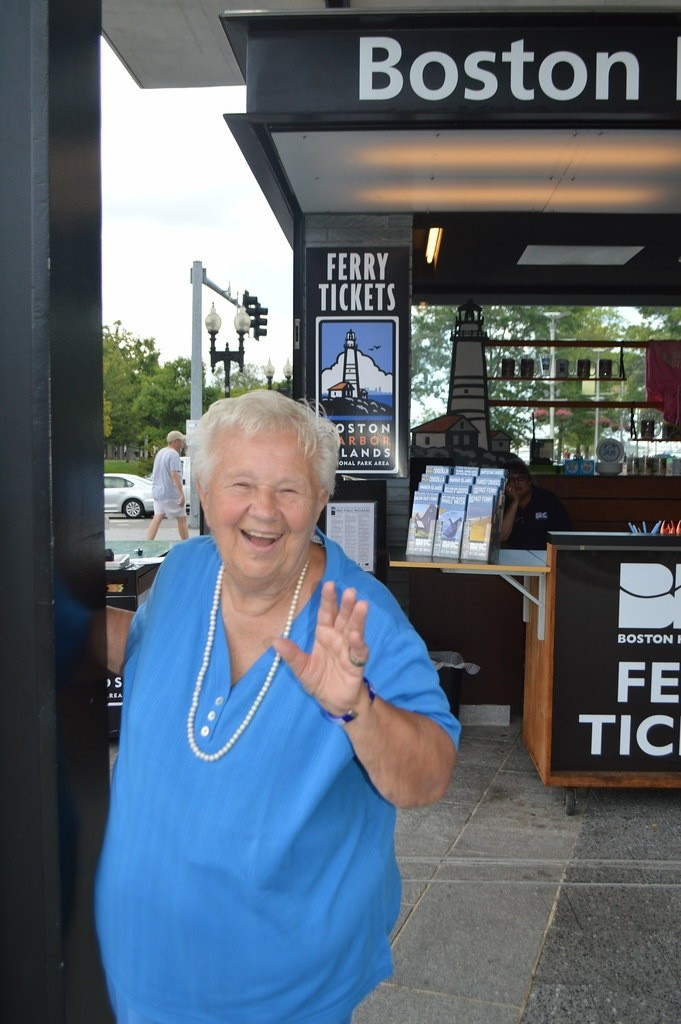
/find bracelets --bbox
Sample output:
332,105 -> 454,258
320,678 -> 376,726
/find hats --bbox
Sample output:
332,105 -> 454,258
167,431 -> 188,443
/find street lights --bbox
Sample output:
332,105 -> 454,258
263,358 -> 293,398
205,299 -> 251,399
542,311 -> 564,463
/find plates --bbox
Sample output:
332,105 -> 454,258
596,438 -> 624,462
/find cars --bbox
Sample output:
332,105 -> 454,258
104,473 -> 154,519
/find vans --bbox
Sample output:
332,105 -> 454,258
146,456 -> 190,508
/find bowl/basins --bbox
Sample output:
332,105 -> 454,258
595,462 -> 623,476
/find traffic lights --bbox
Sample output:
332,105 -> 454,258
254,301 -> 268,341
242,290 -> 258,328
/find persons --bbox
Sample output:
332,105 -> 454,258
94,387 -> 461,1021
148,429 -> 192,540
501,460 -> 573,551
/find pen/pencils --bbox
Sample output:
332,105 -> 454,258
628,520 -> 662,534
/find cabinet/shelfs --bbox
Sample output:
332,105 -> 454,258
482,335 -> 680,477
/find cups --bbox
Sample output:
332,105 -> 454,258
521,359 -> 538,378
672,459 -> 681,476
540,354 -> 553,378
577,359 -> 596,378
502,359 -> 520,377
556,359 -> 575,378
626,456 -> 667,476
641,420 -> 654,440
599,359 -> 619,378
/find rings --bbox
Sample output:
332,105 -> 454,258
349,655 -> 366,667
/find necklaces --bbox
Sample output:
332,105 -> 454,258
186,552 -> 309,762
